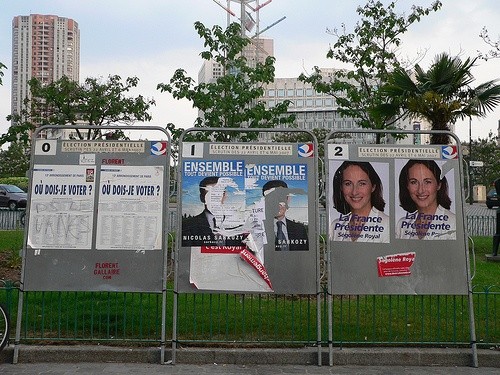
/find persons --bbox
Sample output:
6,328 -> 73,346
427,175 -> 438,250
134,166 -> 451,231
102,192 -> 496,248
263,181 -> 309,250
185,177 -> 245,245
331,161 -> 390,243
397,158 -> 456,240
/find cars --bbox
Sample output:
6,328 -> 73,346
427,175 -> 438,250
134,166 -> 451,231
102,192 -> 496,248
486,188 -> 500,210
0,183 -> 27,211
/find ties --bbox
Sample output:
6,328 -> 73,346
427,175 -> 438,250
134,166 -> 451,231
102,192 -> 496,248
275,221 -> 289,251
213,217 -> 225,247
